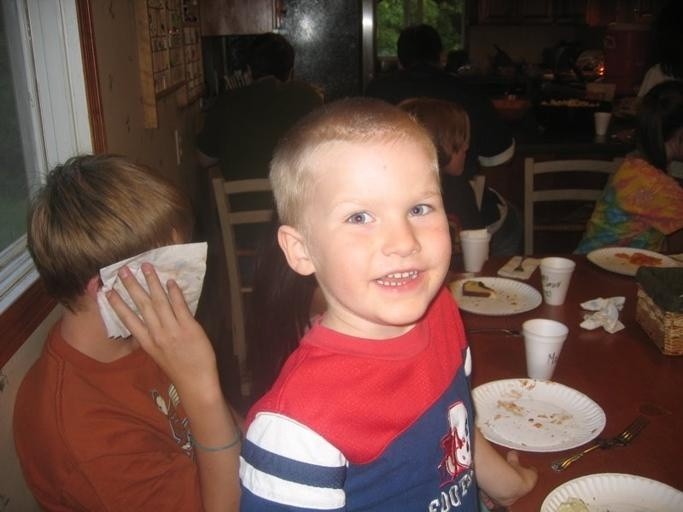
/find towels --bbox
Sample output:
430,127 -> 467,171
97,238 -> 210,344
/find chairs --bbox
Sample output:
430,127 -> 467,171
208,171 -> 286,401
522,154 -> 614,258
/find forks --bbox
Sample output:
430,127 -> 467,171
552,416 -> 648,471
512,256 -> 527,272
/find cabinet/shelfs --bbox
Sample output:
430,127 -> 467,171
132,2 -> 208,131
198,0 -> 286,39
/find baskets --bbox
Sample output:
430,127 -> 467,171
636,268 -> 683,356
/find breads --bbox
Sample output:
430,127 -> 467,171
462,280 -> 492,298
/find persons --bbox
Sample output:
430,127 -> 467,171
238,94 -> 537,511
12,155 -> 247,512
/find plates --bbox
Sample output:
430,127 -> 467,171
470,378 -> 608,452
447,277 -> 544,317
586,248 -> 680,277
541,473 -> 682,511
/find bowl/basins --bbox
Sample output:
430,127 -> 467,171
534,95 -> 613,131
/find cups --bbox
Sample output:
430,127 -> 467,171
521,319 -> 569,379
592,112 -> 610,134
538,256 -> 575,306
459,229 -> 490,272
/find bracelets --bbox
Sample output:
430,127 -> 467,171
190,434 -> 239,452
